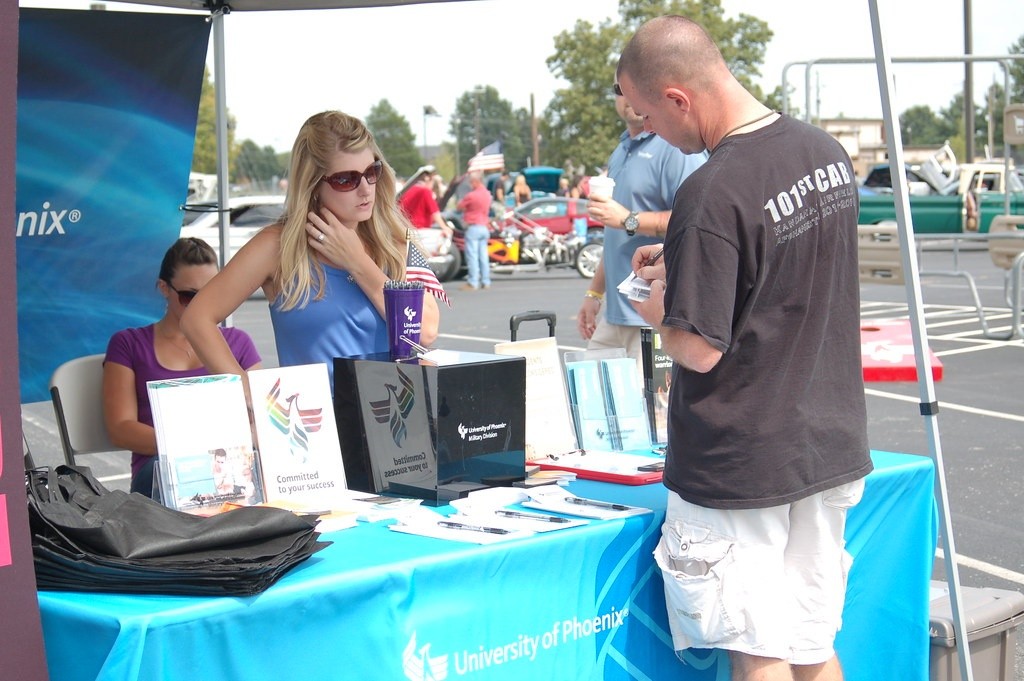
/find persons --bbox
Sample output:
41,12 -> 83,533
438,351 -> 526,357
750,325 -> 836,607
455,172 -> 492,289
212,448 -> 234,494
494,170 -> 510,204
240,463 -> 261,504
179,110 -> 439,411
513,175 -> 531,207
616,16 -> 874,681
103,237 -> 262,498
560,165 -> 608,198
578,72 -> 711,362
397,171 -> 454,237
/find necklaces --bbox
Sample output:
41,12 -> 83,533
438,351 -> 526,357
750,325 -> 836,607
717,110 -> 775,145
157,323 -> 194,359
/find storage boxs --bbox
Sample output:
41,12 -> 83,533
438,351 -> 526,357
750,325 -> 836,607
930,578 -> 1024,681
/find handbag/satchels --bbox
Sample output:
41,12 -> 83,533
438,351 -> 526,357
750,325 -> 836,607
22,464 -> 333,596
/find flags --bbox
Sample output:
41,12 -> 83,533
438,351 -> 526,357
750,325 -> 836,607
467,140 -> 505,172
405,242 -> 451,309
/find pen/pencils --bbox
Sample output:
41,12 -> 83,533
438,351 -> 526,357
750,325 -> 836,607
565,497 -> 631,511
631,248 -> 664,282
495,511 -> 571,523
438,522 -> 509,534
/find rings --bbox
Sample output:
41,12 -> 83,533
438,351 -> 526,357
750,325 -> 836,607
318,233 -> 324,242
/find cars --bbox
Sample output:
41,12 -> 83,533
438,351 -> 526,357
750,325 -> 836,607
855,157 -> 1024,254
428,163 -> 613,285
178,194 -> 291,300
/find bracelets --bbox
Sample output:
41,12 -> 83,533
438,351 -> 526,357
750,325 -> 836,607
584,290 -> 603,306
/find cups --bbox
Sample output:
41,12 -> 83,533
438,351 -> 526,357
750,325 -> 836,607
589,176 -> 615,221
383,288 -> 424,356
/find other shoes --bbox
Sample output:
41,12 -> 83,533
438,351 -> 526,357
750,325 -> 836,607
458,283 -> 475,291
478,282 -> 490,289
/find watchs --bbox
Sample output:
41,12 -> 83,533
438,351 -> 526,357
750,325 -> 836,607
624,211 -> 639,236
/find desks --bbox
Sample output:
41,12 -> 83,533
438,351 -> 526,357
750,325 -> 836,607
36,443 -> 939,681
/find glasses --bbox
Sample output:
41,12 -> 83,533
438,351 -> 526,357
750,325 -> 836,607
614,84 -> 623,95
163,280 -> 194,307
320,160 -> 384,193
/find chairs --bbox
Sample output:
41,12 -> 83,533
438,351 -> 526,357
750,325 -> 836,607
50,353 -> 129,466
